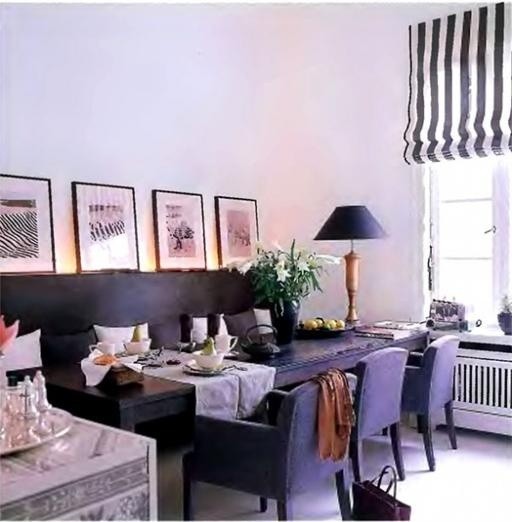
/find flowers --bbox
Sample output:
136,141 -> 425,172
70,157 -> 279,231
224,237 -> 344,319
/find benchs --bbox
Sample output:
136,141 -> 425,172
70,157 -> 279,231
1,270 -> 300,387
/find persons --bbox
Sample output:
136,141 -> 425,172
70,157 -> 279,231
437,303 -> 458,317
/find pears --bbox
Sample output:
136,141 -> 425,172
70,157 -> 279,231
132,326 -> 145,342
202,338 -> 216,356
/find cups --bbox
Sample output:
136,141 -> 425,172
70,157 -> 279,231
214,333 -> 239,353
87,340 -> 116,360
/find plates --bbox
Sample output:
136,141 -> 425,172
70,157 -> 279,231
295,325 -> 357,336
183,362 -> 221,376
1,405 -> 73,455
218,351 -> 240,358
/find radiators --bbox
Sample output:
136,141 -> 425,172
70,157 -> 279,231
409,335 -> 512,443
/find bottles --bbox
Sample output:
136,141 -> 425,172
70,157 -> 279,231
0,369 -> 50,448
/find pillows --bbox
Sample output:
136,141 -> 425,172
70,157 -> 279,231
0,307 -> 282,387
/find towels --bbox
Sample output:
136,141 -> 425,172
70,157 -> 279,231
79,348 -> 139,388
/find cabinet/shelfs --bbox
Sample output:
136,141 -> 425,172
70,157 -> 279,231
0,395 -> 160,521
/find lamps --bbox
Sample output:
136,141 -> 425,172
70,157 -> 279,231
311,205 -> 390,328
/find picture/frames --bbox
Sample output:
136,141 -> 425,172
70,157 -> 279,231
214,195 -> 264,270
72,181 -> 140,272
152,188 -> 207,272
1,174 -> 56,274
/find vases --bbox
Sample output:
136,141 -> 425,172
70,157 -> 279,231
270,290 -> 297,346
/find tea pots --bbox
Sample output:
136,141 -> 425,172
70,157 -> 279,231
239,322 -> 280,359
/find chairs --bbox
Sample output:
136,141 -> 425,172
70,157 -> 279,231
398,332 -> 460,471
340,345 -> 408,487
180,368 -> 361,520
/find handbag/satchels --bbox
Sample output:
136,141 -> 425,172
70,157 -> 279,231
352,481 -> 410,520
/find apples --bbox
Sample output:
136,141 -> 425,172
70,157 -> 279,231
304,318 -> 347,330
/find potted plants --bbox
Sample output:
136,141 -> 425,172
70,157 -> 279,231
497,294 -> 512,336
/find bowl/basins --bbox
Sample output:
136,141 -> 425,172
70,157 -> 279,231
122,337 -> 153,355
190,348 -> 225,370
